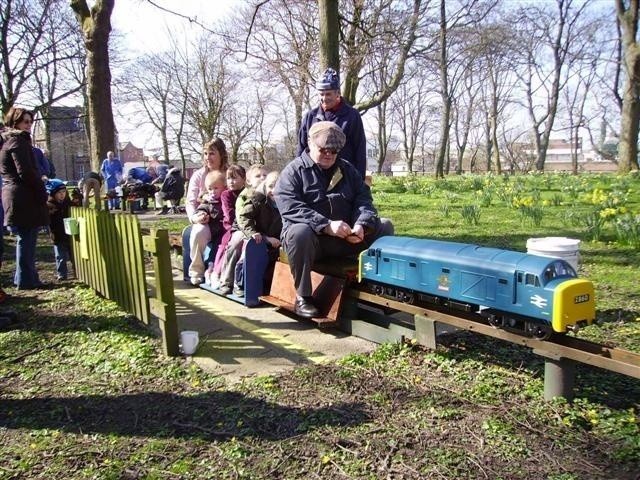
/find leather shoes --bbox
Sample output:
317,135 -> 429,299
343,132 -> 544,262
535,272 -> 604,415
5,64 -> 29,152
294,298 -> 318,318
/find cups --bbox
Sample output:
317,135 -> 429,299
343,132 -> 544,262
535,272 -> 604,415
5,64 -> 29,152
180,330 -> 199,355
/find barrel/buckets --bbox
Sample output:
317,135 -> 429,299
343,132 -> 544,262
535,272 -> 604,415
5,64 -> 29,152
526,235 -> 581,274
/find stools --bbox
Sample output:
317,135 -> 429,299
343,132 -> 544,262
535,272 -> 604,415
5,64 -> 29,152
168,198 -> 181,215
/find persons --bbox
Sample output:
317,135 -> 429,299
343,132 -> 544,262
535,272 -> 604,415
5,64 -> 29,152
44,178 -> 79,279
72,152 -> 184,215
33,146 -> 51,234
296,68 -> 366,183
41,148 -> 56,178
182,137 -> 283,298
0,108 -> 53,290
273,121 -> 393,317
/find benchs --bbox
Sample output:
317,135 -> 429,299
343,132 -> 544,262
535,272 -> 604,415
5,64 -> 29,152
178,236 -> 358,329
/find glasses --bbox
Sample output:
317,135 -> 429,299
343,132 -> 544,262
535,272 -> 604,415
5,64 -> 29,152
319,147 -> 341,154
24,119 -> 33,124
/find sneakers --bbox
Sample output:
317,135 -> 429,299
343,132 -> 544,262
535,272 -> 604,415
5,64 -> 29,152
220,287 -> 230,294
233,287 -> 243,296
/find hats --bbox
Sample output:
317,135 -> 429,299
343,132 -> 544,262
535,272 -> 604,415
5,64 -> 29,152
309,121 -> 345,148
316,68 -> 340,89
44,175 -> 66,195
